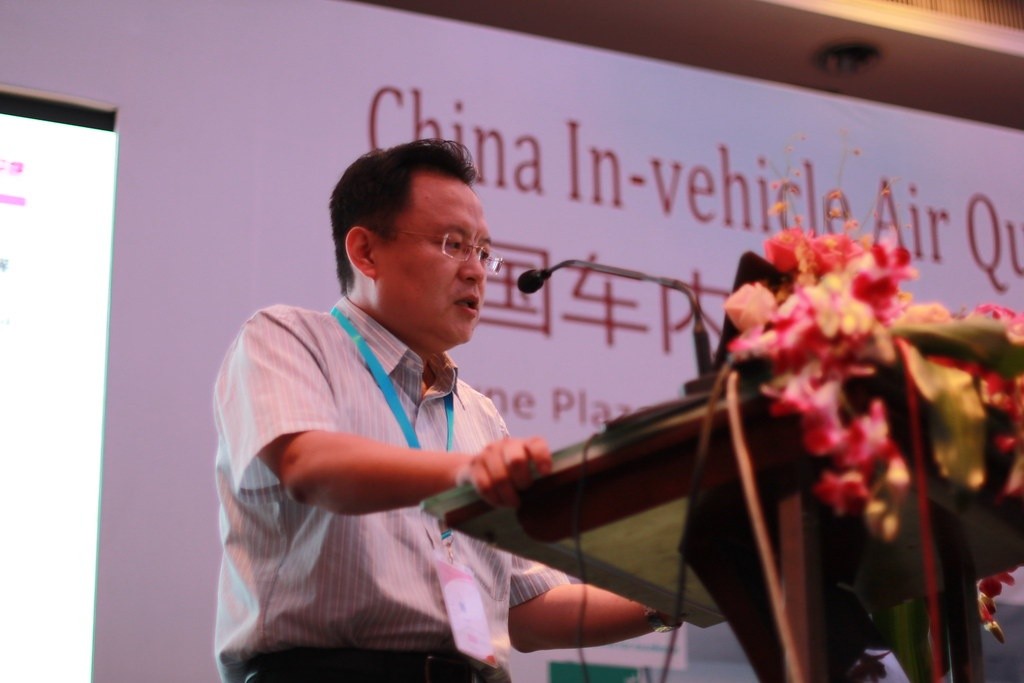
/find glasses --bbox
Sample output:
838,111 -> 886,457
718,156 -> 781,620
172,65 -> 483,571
380,228 -> 505,276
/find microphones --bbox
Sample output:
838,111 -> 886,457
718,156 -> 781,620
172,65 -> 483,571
518,260 -> 716,396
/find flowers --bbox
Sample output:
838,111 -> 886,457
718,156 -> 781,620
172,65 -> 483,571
724,113 -> 1023,643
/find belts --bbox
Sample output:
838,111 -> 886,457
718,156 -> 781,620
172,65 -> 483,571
245,652 -> 485,683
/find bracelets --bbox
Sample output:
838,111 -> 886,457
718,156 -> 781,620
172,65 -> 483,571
643,602 -> 682,633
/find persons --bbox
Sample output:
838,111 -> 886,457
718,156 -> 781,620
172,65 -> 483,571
212,137 -> 684,683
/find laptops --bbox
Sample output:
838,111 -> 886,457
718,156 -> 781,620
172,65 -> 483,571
597,251 -> 794,434
